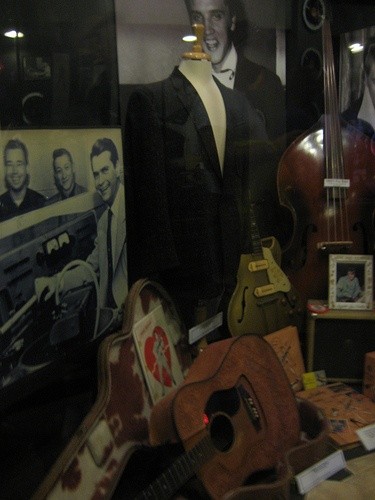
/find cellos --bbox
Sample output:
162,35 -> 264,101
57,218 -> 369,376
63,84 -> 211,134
279,0 -> 375,385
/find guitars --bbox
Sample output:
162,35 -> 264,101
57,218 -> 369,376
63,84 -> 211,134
226,169 -> 305,338
132,331 -> 301,500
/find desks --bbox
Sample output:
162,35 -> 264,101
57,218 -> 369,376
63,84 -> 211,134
305,298 -> 375,374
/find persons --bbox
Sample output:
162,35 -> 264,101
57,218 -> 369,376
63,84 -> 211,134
0,139 -> 46,223
186,0 -> 284,164
33,138 -> 128,314
44,148 -> 87,205
335,267 -> 361,302
124,23 -> 249,315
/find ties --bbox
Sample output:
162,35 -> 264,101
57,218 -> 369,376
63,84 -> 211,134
103,210 -> 118,310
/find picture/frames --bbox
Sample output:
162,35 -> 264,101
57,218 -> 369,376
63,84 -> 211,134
328,254 -> 374,311
